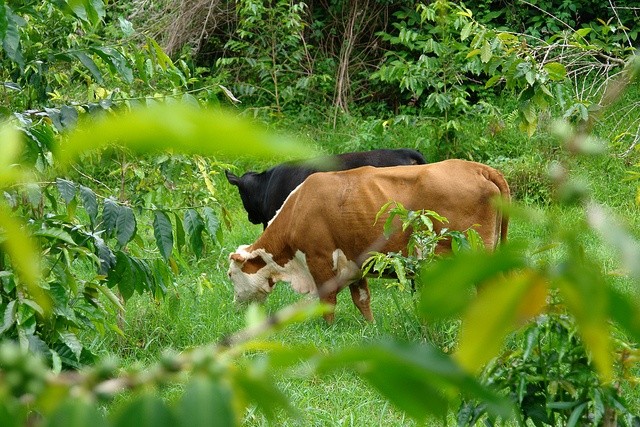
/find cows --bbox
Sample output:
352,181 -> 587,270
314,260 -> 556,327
225,149 -> 427,280
225,158 -> 512,328
